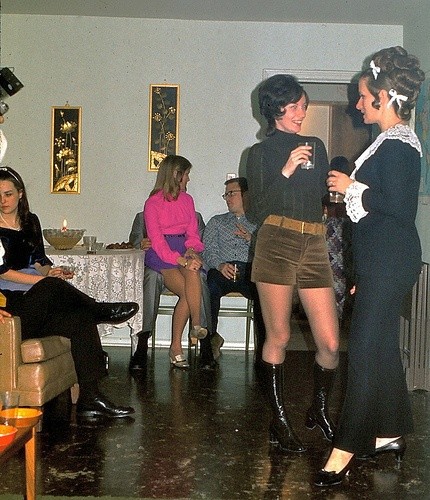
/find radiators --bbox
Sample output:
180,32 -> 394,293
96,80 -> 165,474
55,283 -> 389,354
399,262 -> 430,391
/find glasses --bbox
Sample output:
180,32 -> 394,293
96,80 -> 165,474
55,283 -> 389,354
222,189 -> 244,201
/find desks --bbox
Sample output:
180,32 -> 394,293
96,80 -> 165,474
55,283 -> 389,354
46,245 -> 145,356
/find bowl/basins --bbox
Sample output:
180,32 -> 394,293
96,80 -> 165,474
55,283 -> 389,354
0,407 -> 43,439
43,228 -> 88,250
0,426 -> 18,454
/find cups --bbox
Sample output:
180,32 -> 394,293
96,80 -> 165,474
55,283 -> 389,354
330,190 -> 344,203
298,141 -> 316,170
84,236 -> 104,255
0,391 -> 19,435
230,264 -> 240,283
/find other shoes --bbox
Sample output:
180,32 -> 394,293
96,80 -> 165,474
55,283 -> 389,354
199,355 -> 220,372
210,331 -> 225,360
133,348 -> 148,367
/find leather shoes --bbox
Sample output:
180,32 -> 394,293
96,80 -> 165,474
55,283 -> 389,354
96,301 -> 139,325
75,393 -> 136,418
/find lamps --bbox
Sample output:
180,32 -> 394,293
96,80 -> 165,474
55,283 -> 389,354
0,66 -> 23,97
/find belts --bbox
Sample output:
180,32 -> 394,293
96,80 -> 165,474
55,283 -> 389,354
263,214 -> 329,236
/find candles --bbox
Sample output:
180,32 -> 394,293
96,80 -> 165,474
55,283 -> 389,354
61,219 -> 67,232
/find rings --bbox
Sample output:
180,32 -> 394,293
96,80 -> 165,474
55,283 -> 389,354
244,232 -> 247,234
332,182 -> 336,186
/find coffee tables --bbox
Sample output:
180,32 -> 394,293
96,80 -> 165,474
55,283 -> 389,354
0,418 -> 42,500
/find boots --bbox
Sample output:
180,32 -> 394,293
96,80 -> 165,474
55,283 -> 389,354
258,358 -> 308,453
304,360 -> 337,442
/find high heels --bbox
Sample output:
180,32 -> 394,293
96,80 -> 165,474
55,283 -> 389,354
167,350 -> 191,368
189,325 -> 208,345
356,435 -> 407,463
313,450 -> 357,487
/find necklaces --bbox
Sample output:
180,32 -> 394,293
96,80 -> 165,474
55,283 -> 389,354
0,212 -> 12,228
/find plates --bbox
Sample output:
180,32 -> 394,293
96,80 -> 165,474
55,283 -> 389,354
106,249 -> 135,251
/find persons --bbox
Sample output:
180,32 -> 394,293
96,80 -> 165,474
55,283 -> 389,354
0,167 -> 139,417
130,211 -> 219,370
202,177 -> 264,360
327,156 -> 350,217
314,46 -> 425,487
250,75 -> 340,451
144,154 -> 207,369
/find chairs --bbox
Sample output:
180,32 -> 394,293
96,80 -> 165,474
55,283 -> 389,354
149,291 -> 258,357
0,316 -> 79,431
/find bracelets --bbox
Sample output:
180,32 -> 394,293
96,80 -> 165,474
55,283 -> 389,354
184,259 -> 188,268
192,248 -> 196,252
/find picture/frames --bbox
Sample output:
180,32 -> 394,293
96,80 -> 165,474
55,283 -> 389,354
149,84 -> 179,171
50,105 -> 82,194
